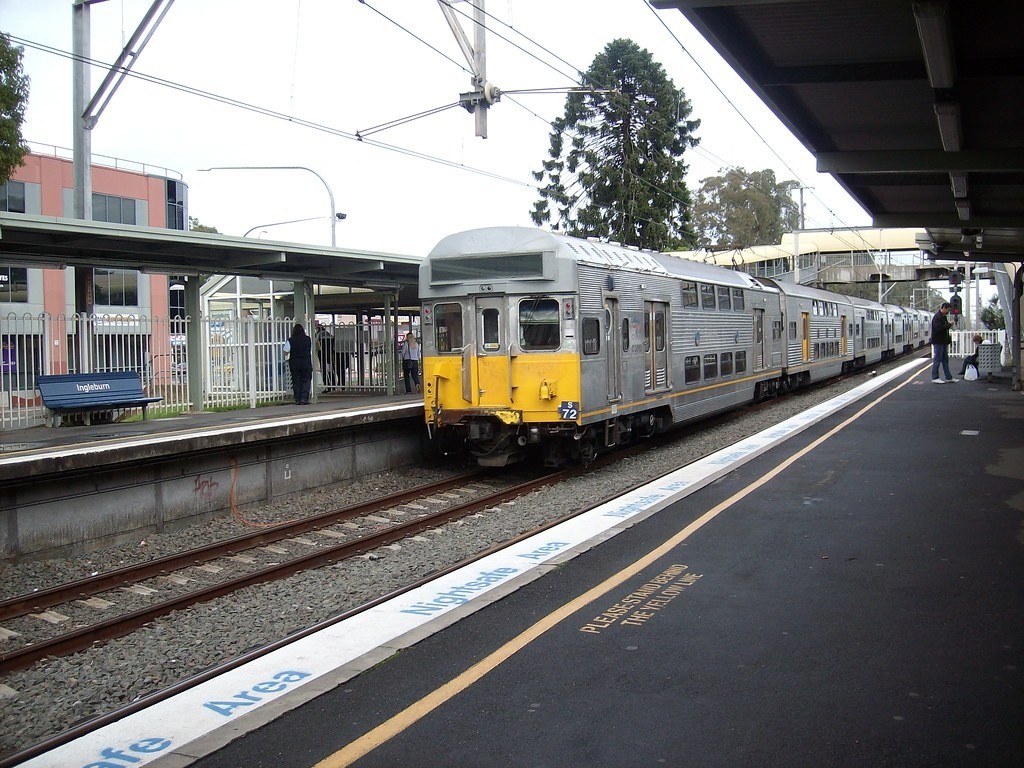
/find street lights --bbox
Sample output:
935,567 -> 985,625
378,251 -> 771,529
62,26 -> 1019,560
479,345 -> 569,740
970,267 -> 1013,325
234,211 -> 348,320
195,162 -> 339,251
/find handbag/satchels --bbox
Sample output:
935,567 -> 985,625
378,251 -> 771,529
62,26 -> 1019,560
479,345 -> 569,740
963,364 -> 978,381
404,361 -> 413,370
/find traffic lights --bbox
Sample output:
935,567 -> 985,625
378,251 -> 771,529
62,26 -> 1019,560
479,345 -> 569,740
949,271 -> 961,284
949,295 -> 964,315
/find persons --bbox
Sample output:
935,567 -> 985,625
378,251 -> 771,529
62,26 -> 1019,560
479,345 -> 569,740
931,303 -> 960,384
315,324 -> 349,393
283,324 -> 313,405
957,335 -> 991,375
402,333 -> 421,394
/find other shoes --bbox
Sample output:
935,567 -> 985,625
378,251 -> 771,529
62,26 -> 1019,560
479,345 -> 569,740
296,400 -> 308,405
404,389 -> 411,393
945,376 -> 960,383
322,388 -> 330,393
958,372 -> 964,375
416,384 -> 421,393
932,377 -> 946,383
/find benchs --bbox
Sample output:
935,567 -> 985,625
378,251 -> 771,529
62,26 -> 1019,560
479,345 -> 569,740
35,372 -> 164,428
976,346 -> 1003,380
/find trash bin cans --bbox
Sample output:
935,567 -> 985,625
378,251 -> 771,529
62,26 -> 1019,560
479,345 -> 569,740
978,344 -> 1001,378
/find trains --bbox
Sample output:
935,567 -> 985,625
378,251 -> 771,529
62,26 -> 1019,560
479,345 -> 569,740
416,227 -> 938,474
